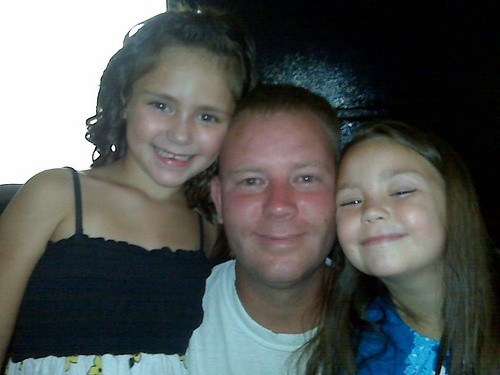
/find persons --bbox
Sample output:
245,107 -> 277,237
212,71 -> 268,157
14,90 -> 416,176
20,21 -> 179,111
0,5 -> 253,373
180,82 -> 361,374
304,120 -> 500,375
